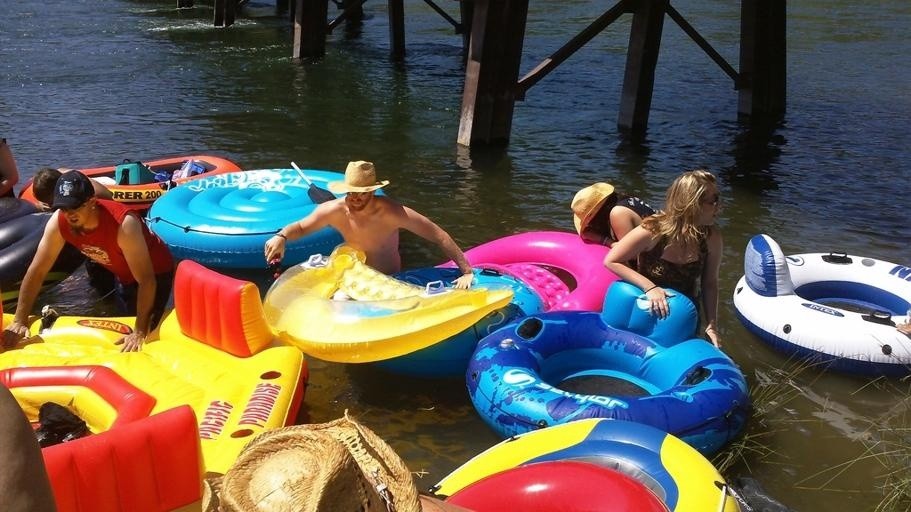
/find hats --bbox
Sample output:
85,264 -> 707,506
569,180 -> 614,237
203,411 -> 422,512
325,160 -> 392,195
47,169 -> 97,211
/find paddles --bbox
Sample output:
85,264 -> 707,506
288,161 -> 338,205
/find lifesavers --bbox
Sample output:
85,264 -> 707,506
465,279 -> 752,453
733,232 -> 911,374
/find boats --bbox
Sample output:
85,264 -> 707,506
0,260 -> 307,512
18,155 -> 243,212
146,167 -> 386,271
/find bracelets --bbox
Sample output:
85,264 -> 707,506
275,232 -> 288,240
645,284 -> 659,293
602,237 -> 608,245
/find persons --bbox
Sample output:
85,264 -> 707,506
3,171 -> 176,351
32,168 -> 113,286
1,138 -> 18,196
264,160 -> 473,291
604,170 -> 723,349
571,182 -> 658,260
0,381 -> 58,512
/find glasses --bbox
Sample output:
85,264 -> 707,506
57,205 -> 82,211
701,191 -> 721,205
